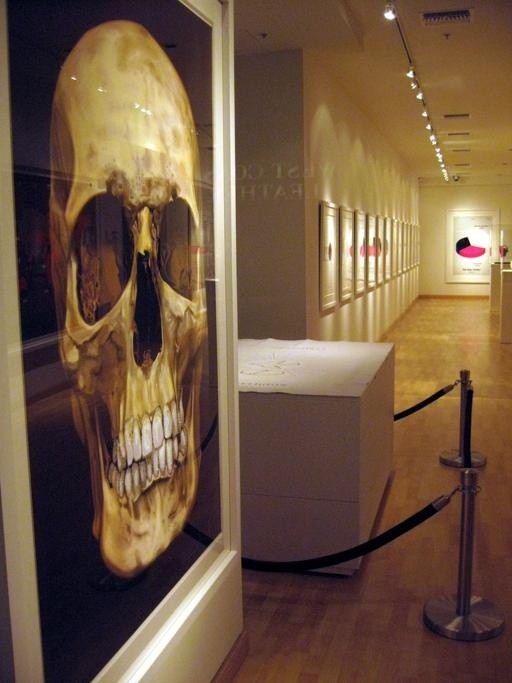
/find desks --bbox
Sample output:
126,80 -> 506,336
239,335 -> 399,578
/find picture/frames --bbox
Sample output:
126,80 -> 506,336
444,207 -> 503,285
319,199 -> 421,320
